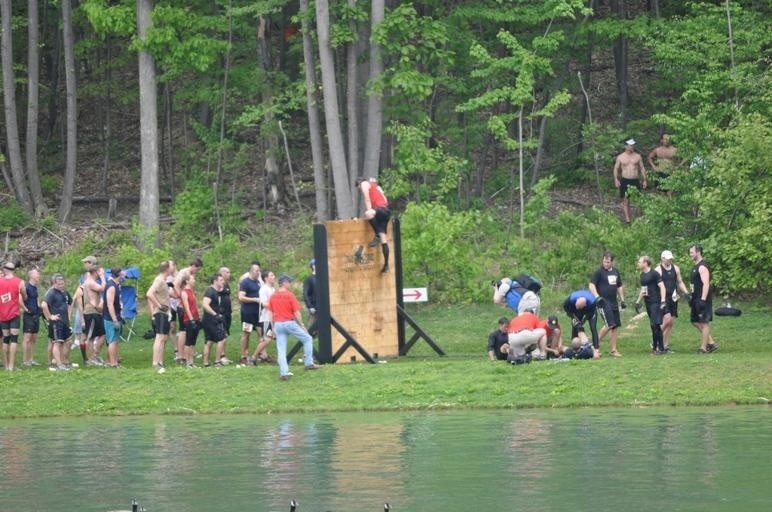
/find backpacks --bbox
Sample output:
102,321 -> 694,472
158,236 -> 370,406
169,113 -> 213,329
514,273 -> 544,293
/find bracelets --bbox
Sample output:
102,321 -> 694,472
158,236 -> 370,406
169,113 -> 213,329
642,175 -> 647,181
614,175 -> 618,179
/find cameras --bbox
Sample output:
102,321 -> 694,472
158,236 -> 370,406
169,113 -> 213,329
491,280 -> 502,287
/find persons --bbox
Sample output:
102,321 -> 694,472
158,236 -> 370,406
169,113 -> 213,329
485,242 -> 720,365
354,174 -> 392,275
268,274 -> 322,380
647,133 -> 689,199
612,137 -> 647,226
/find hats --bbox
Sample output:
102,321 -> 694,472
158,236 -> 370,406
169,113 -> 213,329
82,255 -> 97,263
546,315 -> 559,328
661,251 -> 673,260
625,138 -> 637,145
277,274 -> 293,282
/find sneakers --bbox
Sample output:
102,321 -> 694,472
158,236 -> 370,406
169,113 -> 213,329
381,265 -> 390,272
590,342 -> 722,356
368,235 -> 382,247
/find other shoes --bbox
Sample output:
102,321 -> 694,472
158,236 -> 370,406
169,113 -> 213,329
303,363 -> 320,369
48,358 -> 125,371
280,372 -> 294,377
152,363 -> 165,374
172,356 -> 274,368
5,360 -> 40,371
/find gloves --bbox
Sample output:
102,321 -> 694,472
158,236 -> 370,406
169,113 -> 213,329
596,297 -> 711,310
190,320 -> 201,329
